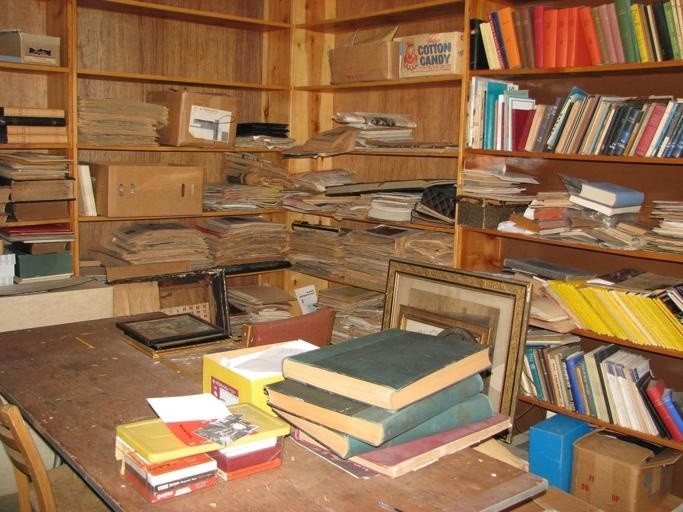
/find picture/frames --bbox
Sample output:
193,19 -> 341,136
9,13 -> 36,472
381,258 -> 533,445
116,313 -> 232,359
397,305 -> 493,348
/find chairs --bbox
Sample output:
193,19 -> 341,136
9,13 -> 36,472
0,404 -> 113,512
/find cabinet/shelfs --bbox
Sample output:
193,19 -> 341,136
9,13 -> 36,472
283,0 -> 465,297
0,0 -> 78,288
458,1 -> 683,450
78,2 -> 293,266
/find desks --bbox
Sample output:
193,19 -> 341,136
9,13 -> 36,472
1,311 -> 548,511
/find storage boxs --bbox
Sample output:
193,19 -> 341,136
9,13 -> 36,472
394,30 -> 461,74
328,26 -> 399,84
527,415 -> 593,492
4,248 -> 72,278
572,427 -> 682,512
81,163 -> 204,219
3,30 -> 61,66
160,276 -> 217,323
151,89 -> 238,149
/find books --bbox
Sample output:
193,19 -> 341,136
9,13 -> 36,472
456,166 -> 683,256
467,76 -> 682,159
234,121 -> 298,150
79,163 -> 98,220
0,26 -> 63,66
493,251 -> 682,444
316,285 -> 386,347
300,112 -> 459,159
466,0 -> 683,70
0,225 -> 75,285
2,107 -> 68,145
291,413 -> 513,480
281,327 -> 492,412
1,151 -> 77,222
78,210 -> 288,268
78,97 -> 172,148
289,214 -> 454,291
264,373 -> 485,448
325,175 -> 457,227
270,393 -> 494,459
227,273 -> 318,341
203,151 -> 362,223
113,401 -> 282,504
